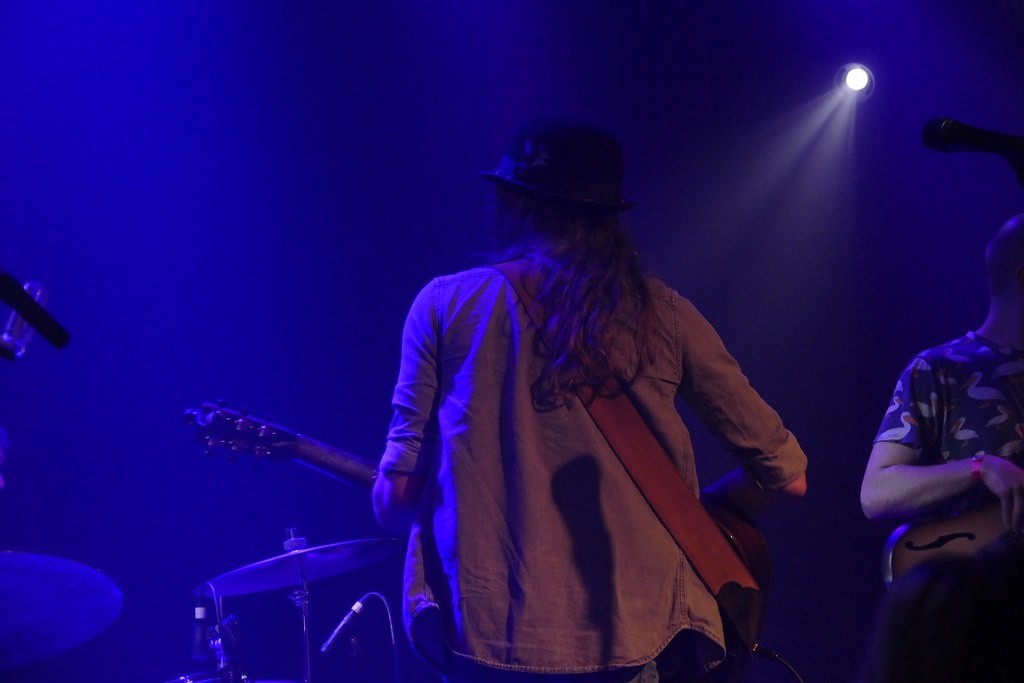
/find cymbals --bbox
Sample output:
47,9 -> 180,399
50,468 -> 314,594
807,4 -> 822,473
190,531 -> 402,601
1,549 -> 127,676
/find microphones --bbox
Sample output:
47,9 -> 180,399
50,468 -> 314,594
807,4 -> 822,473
0,267 -> 70,348
921,118 -> 1024,159
321,595 -> 370,654
192,591 -> 210,659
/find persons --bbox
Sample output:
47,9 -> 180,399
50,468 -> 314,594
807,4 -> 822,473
859,213 -> 1024,683
370,120 -> 807,683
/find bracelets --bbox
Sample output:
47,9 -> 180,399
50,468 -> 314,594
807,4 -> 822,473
973,451 -> 985,481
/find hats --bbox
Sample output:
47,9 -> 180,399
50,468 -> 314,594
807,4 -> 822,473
480,118 -> 630,216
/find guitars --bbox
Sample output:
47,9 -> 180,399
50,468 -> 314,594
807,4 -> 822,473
181,399 -> 769,683
878,487 -> 1024,594
